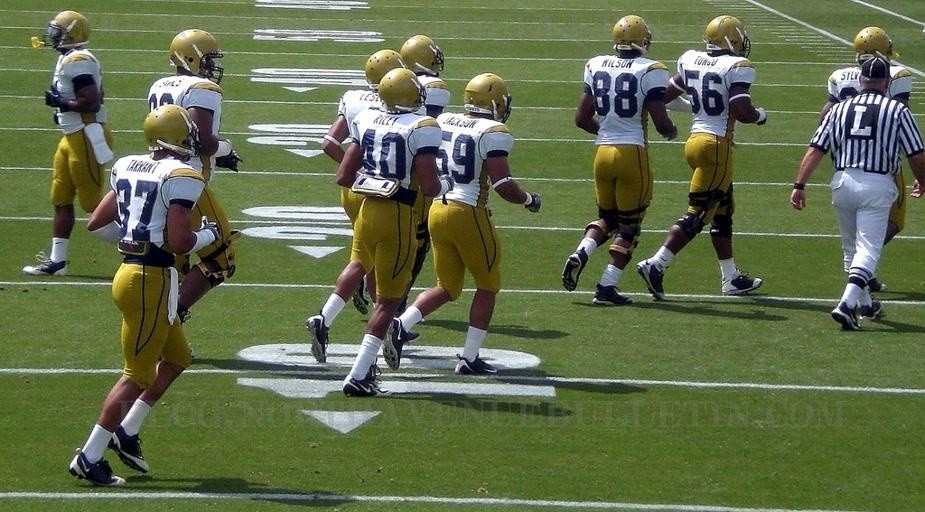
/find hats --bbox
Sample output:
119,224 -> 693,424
860,56 -> 892,79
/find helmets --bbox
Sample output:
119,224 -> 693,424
611,15 -> 652,55
378,67 -> 422,112
142,103 -> 196,158
46,10 -> 92,50
703,15 -> 748,56
463,72 -> 510,121
169,28 -> 221,78
854,26 -> 894,62
364,49 -> 408,89
399,34 -> 440,77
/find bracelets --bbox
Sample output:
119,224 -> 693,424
793,182 -> 805,190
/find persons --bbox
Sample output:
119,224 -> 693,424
308,36 -> 540,397
147,29 -> 236,363
21,9 -> 112,278
818,28 -> 912,293
637,15 -> 767,301
67,105 -> 218,487
792,56 -> 925,332
563,15 -> 678,306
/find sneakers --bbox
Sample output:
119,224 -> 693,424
106,423 -> 151,474
342,374 -> 393,398
560,246 -> 589,292
351,274 -> 369,316
591,282 -> 635,306
366,358 -> 381,380
382,316 -> 420,371
831,300 -> 862,332
721,270 -> 763,295
67,447 -> 129,488
869,278 -> 888,292
305,310 -> 330,365
859,300 -> 883,321
454,353 -> 499,375
636,257 -> 666,301
20,251 -> 71,278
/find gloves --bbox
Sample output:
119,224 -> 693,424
215,150 -> 244,172
752,107 -> 767,126
44,86 -> 70,109
524,190 -> 542,213
193,215 -> 222,245
439,174 -> 455,193
